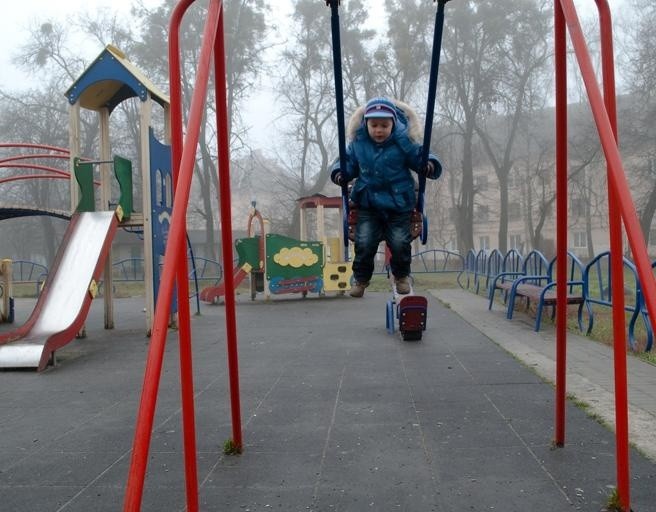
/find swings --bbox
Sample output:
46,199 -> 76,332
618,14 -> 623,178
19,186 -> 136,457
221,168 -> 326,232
324,0 -> 448,247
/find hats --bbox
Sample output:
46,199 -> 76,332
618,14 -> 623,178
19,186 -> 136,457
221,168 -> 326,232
363,97 -> 398,126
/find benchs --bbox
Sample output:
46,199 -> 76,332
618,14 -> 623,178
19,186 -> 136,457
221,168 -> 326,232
486,248 -> 585,333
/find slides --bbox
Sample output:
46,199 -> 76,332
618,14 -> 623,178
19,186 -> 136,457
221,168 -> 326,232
200,266 -> 249,303
1,210 -> 119,373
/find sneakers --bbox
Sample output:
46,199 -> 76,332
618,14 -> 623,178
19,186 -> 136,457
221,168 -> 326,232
395,275 -> 410,294
350,280 -> 370,297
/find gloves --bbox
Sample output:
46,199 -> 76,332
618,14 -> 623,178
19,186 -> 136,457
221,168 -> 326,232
426,160 -> 434,177
334,171 -> 342,185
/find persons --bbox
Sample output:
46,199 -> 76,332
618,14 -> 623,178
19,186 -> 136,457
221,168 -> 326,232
326,96 -> 441,299
